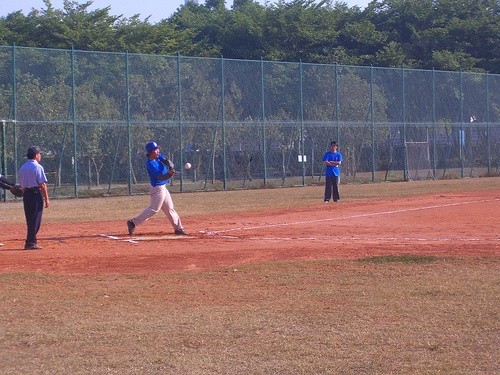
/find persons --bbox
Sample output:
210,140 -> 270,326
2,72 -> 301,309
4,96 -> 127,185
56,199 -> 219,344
126,142 -> 190,236
18,145 -> 50,250
322,141 -> 344,203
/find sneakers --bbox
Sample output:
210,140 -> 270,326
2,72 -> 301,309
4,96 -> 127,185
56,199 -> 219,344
25,243 -> 42,249
126,218 -> 136,234
175,229 -> 189,235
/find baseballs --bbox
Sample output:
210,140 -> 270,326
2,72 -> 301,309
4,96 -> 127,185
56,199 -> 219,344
185,163 -> 191,169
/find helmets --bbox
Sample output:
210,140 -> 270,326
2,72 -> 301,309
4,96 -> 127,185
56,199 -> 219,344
26,145 -> 40,155
146,141 -> 160,152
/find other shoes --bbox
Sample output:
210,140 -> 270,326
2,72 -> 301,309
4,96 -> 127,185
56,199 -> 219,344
334,200 -> 340,203
324,200 -> 329,203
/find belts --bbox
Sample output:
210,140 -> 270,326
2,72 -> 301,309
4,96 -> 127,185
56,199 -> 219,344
23,186 -> 41,192
